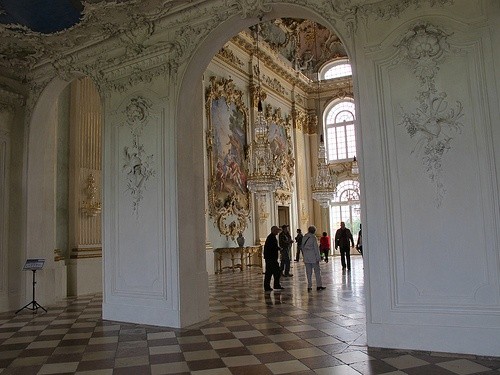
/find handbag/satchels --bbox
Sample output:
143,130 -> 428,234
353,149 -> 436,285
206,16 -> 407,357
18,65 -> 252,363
336,236 -> 341,246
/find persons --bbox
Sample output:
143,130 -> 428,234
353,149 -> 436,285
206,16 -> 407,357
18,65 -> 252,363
302,225 -> 326,291
264,226 -> 285,291
233,192 -> 243,210
320,232 -> 330,263
294,229 -> 303,261
279,225 -> 293,276
356,223 -> 363,254
335,222 -> 354,270
279,153 -> 290,190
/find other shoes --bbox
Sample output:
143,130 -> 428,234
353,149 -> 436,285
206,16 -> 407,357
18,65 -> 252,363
294,260 -> 298,262
274,287 -> 285,289
280,271 -> 285,277
285,273 -> 293,277
342,264 -> 346,270
308,288 -> 312,291
265,288 -> 273,291
317,287 -> 326,290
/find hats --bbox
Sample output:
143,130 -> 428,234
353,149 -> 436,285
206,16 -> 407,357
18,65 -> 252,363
271,226 -> 281,233
282,224 -> 289,229
